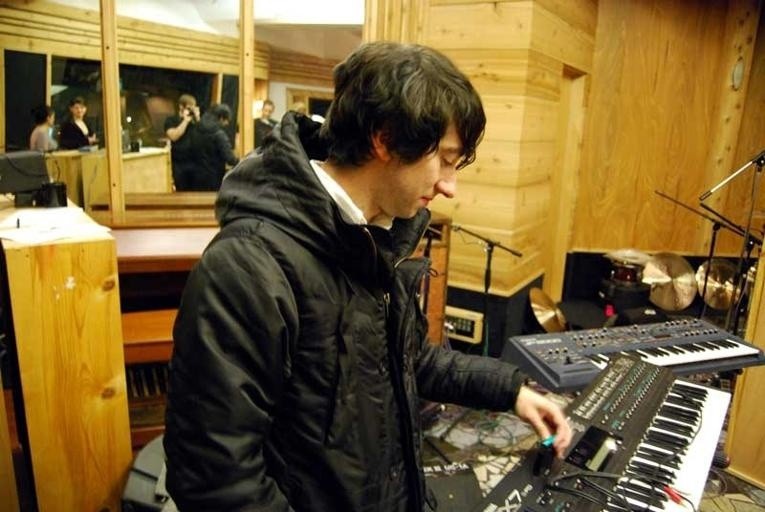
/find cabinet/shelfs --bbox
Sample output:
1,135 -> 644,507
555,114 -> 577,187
0,193 -> 134,512
404,211 -> 451,426
111,226 -> 222,445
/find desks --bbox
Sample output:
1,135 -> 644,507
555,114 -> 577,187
46,138 -> 229,230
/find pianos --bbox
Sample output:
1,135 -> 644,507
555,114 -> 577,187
475,352 -> 732,512
505,317 -> 765,394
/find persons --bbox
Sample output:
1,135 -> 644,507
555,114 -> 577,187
162,93 -> 201,191
162,39 -> 573,511
179,103 -> 243,189
59,95 -> 101,150
27,105 -> 59,154
254,97 -> 278,150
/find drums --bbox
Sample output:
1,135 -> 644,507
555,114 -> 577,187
605,250 -> 650,287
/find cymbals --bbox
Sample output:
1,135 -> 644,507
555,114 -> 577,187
642,252 -> 697,312
695,258 -> 741,309
529,287 -> 568,333
